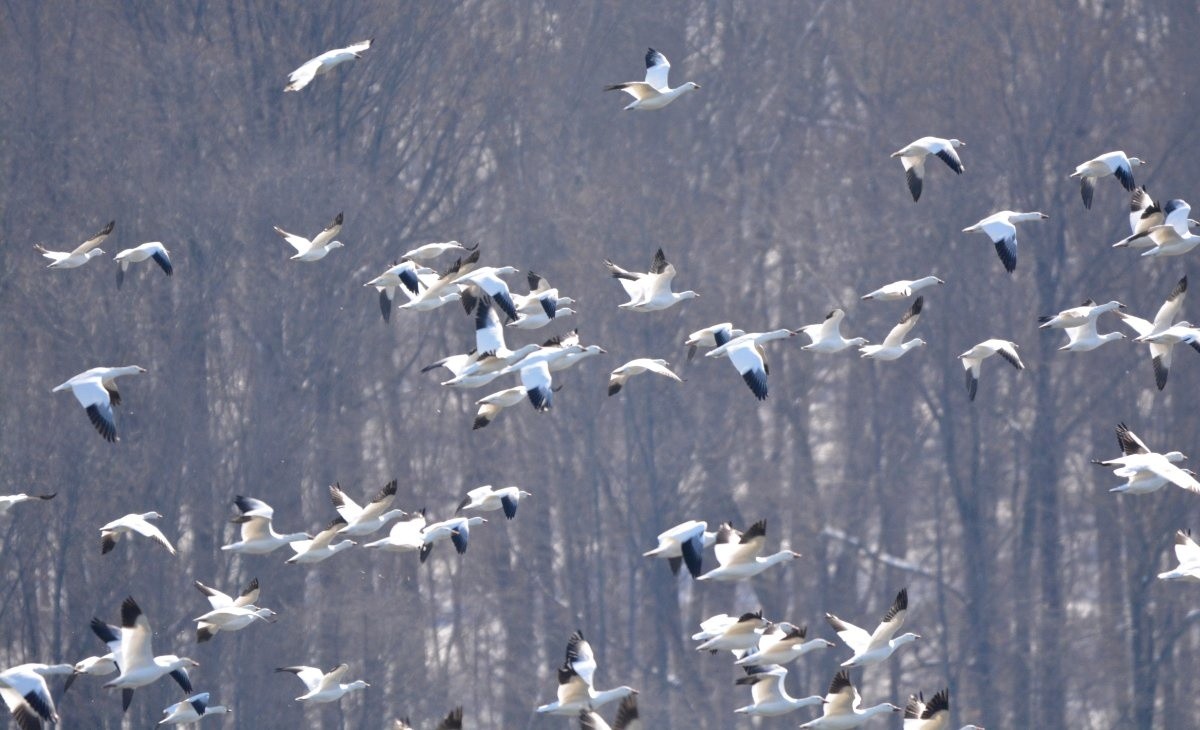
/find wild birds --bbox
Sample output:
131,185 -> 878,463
282,39 -> 374,91
891,130 -> 1200,587
0,470 -> 530,727
36,220 -> 175,286
267,213 -> 946,432
601,48 -> 702,109
53,364 -> 149,443
524,514 -> 952,730
